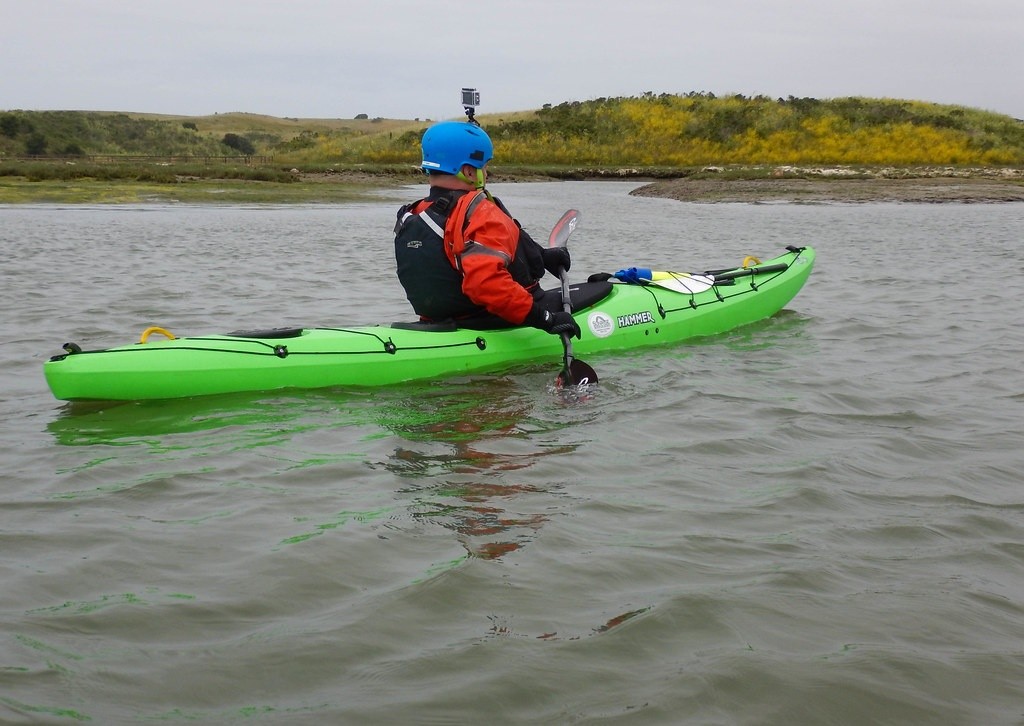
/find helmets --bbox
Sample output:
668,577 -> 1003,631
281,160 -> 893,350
421,120 -> 493,175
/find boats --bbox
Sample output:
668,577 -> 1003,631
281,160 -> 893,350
43,245 -> 818,402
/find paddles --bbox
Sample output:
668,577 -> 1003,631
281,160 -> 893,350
639,262 -> 788,295
549,208 -> 598,405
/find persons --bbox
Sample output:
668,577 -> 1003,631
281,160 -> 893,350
394,120 -> 583,342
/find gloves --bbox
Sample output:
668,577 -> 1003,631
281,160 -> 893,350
542,247 -> 570,279
541,309 -> 580,340
